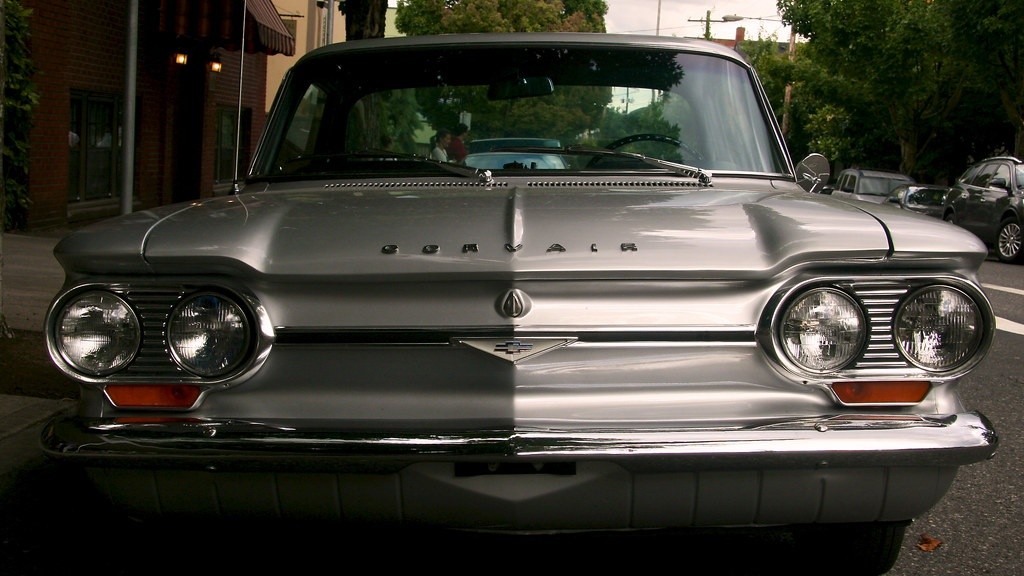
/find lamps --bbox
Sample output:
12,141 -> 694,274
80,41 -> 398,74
173,43 -> 188,65
208,52 -> 223,73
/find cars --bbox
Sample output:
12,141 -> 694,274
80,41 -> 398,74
817,180 -> 837,195
33,30 -> 1001,576
879,183 -> 953,221
470,137 -> 562,159
461,152 -> 570,176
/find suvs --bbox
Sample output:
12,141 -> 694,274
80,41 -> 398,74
942,155 -> 1024,263
830,166 -> 917,206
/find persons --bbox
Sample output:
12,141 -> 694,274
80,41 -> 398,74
381,135 -> 395,152
448,123 -> 469,166
429,127 -> 452,165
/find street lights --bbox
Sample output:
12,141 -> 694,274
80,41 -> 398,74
722,13 -> 797,144
